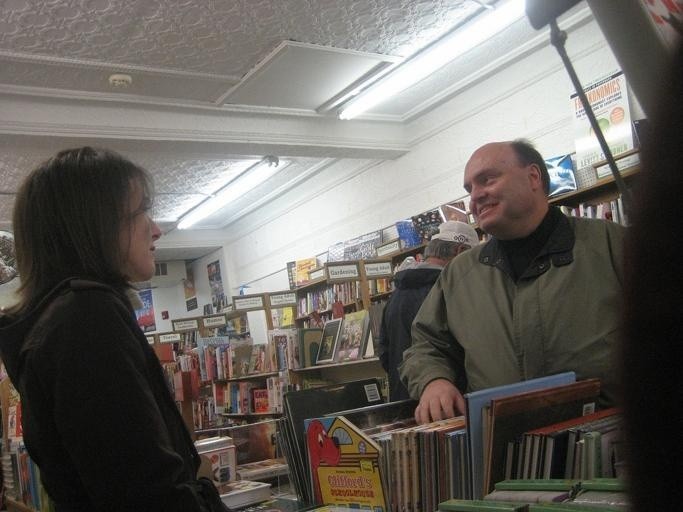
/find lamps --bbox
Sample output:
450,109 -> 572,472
335,1 -> 529,123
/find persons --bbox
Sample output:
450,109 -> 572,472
397,142 -> 625,425
0,148 -> 229,512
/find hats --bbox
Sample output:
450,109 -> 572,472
430,221 -> 480,248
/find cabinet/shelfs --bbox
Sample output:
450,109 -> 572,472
291,256 -> 396,394
547,164 -> 639,228
157,291 -> 291,436
